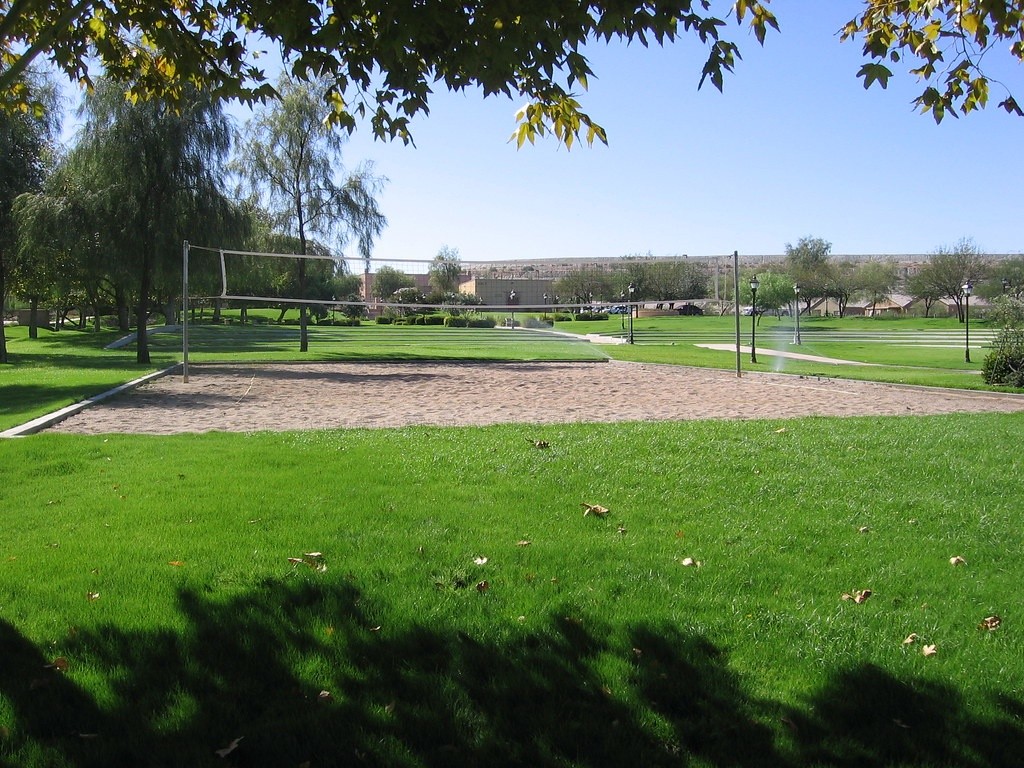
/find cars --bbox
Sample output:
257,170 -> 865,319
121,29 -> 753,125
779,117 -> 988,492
676,305 -> 703,317
592,305 -> 627,314
740,304 -> 795,318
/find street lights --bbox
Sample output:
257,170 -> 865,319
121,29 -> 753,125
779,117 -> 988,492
332,295 -> 336,324
399,295 -> 402,318
628,283 -> 637,345
963,281 -> 972,362
510,290 -> 516,329
621,290 -> 627,328
556,295 -> 561,313
590,292 -> 596,318
749,275 -> 759,362
793,283 -> 803,345
543,293 -> 548,319
452,293 -> 455,313
422,294 -> 427,316
